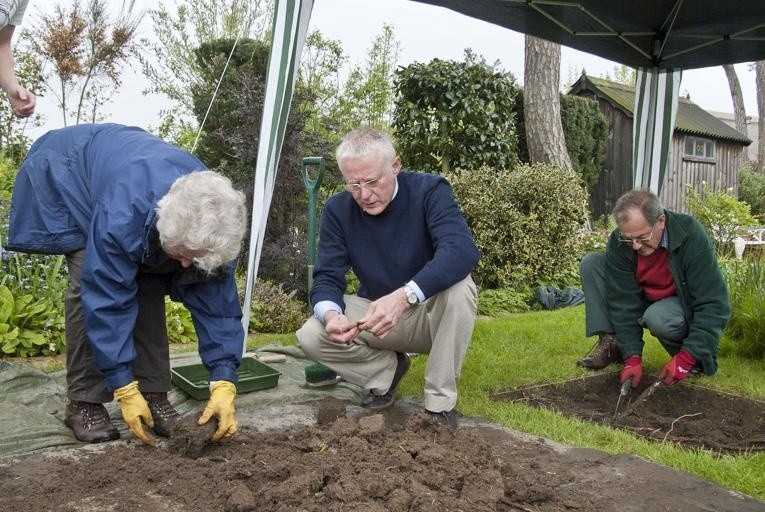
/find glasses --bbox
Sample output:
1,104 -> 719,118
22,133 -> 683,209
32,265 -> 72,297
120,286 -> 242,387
342,175 -> 382,193
618,226 -> 655,245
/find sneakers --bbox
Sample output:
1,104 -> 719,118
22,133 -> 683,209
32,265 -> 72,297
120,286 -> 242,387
65,403 -> 121,443
360,351 -> 411,410
577,333 -> 622,369
424,408 -> 452,427
140,392 -> 183,437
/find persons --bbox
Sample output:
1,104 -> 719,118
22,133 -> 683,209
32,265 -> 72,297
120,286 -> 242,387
1,0 -> 36,119
6,121 -> 249,446
575,186 -> 732,389
295,128 -> 478,429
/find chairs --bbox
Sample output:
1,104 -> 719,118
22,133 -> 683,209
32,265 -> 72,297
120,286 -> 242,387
704,207 -> 765,261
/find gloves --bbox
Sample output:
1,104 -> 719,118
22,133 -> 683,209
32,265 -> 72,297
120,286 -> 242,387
113,380 -> 155,445
619,354 -> 644,389
658,348 -> 696,386
198,381 -> 238,443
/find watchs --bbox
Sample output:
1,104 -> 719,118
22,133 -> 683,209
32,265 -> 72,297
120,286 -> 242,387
401,284 -> 418,310
